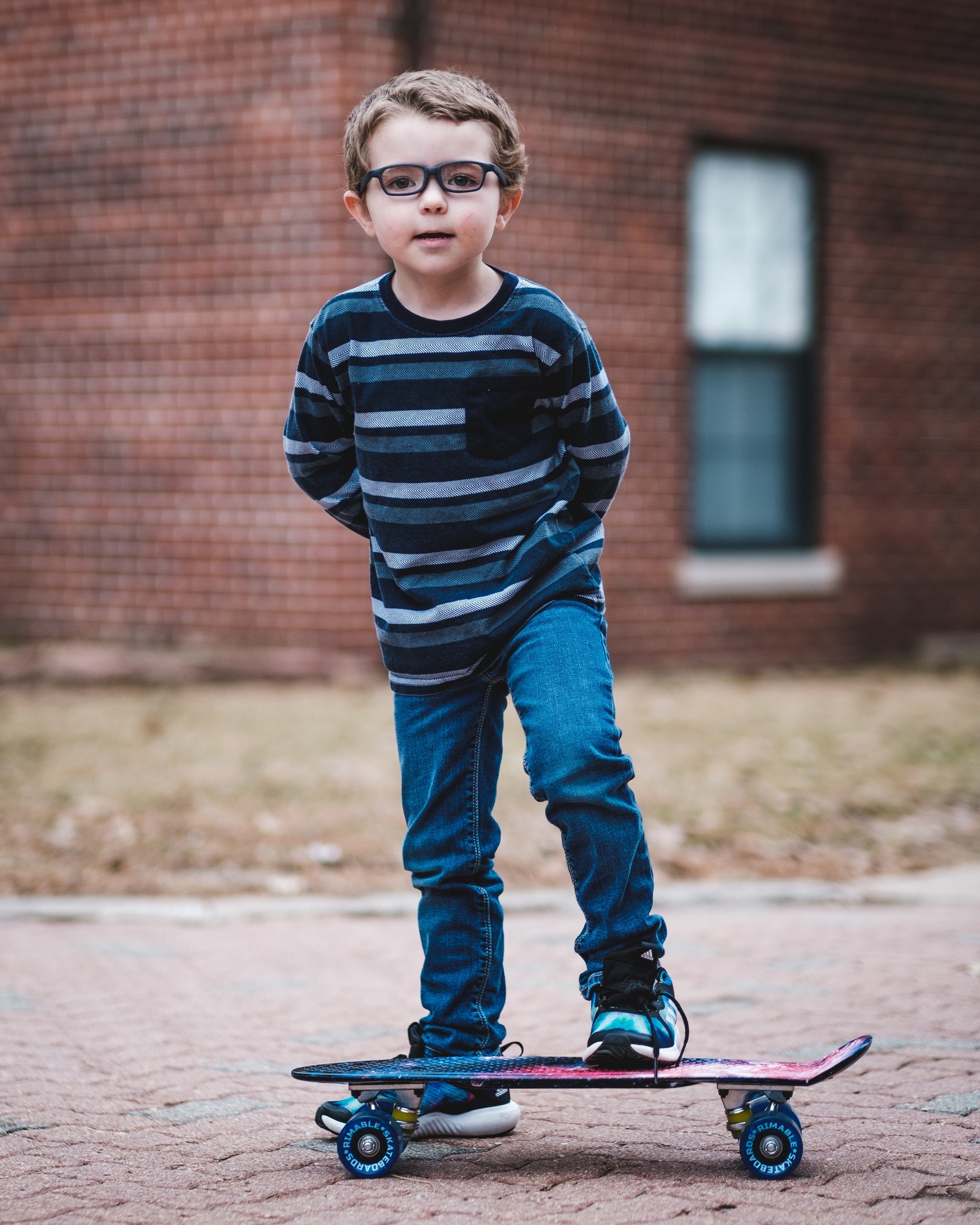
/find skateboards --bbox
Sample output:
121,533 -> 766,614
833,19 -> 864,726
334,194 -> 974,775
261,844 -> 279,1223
292,1035 -> 873,1180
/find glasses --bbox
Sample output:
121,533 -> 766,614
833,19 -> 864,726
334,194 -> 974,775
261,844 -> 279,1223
354,160 -> 509,196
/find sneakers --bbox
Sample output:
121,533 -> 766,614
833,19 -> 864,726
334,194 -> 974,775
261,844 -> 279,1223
315,1089 -> 522,1142
587,949 -> 681,1068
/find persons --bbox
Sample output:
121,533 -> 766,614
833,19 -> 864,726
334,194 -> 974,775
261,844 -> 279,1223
283,68 -> 690,1137
